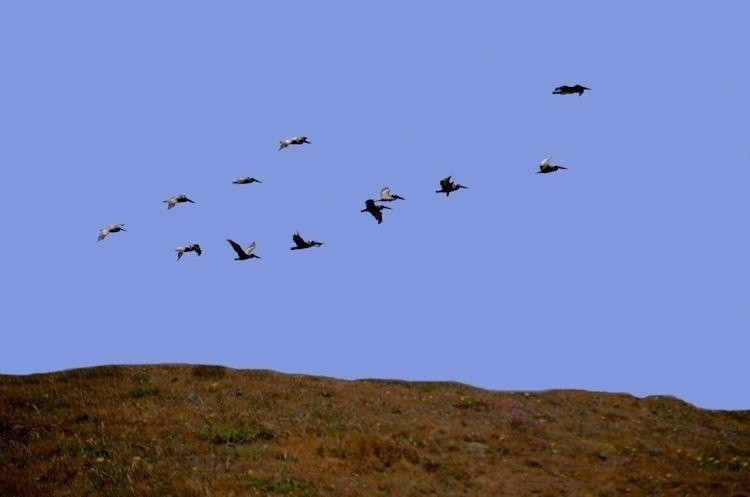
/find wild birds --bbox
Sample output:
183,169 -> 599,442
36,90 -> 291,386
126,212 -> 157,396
95,224 -> 128,242
231,176 -> 263,184
278,136 -> 312,151
162,194 -> 196,210
226,239 -> 260,261
551,85 -> 592,96
290,231 -> 324,250
175,243 -> 202,262
361,187 -> 406,224
435,175 -> 469,197
534,154 -> 567,174
